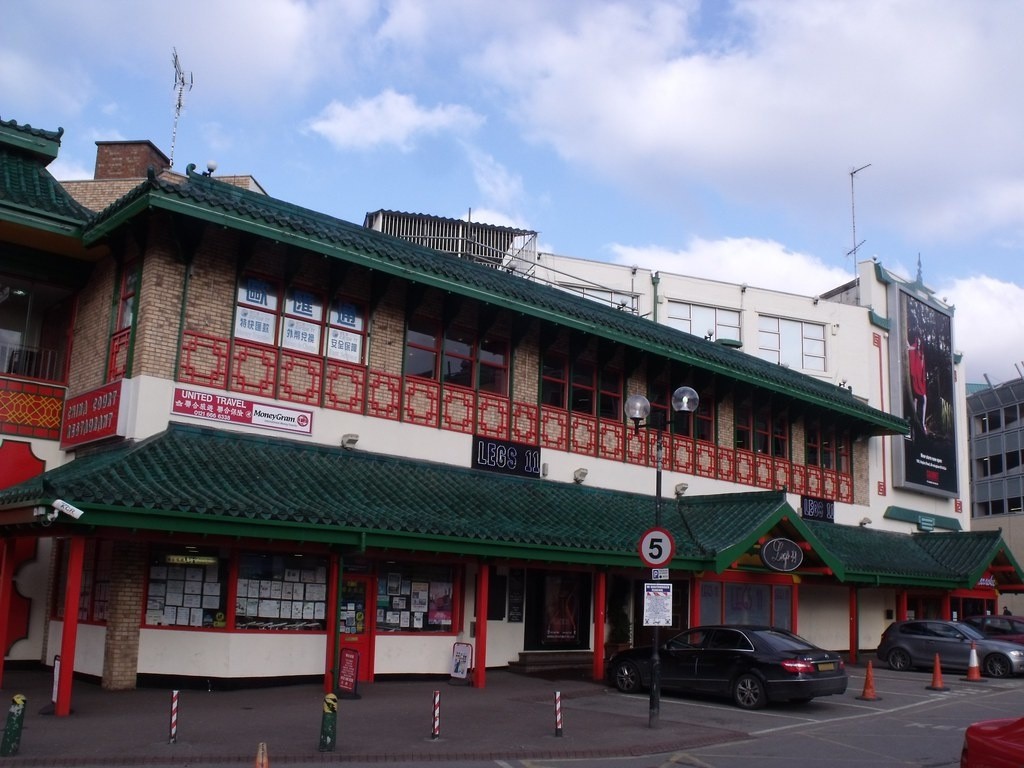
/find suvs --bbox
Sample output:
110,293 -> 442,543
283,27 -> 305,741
876,619 -> 1024,681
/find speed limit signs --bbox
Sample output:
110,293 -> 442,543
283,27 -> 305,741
639,528 -> 674,568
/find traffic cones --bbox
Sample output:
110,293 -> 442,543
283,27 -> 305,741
855,659 -> 882,701
959,640 -> 988,682
926,651 -> 951,690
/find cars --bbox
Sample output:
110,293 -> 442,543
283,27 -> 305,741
964,609 -> 1024,644
960,710 -> 1024,768
608,626 -> 849,709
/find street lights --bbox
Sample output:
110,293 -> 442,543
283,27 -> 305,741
622,384 -> 698,728
